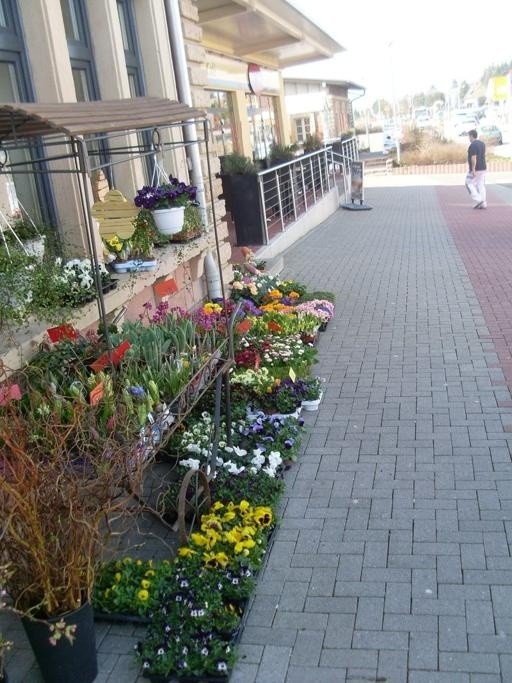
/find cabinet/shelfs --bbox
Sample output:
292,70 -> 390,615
0,98 -> 232,542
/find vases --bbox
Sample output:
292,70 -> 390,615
149,206 -> 188,236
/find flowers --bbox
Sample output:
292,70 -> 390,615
92,252 -> 334,682
134,174 -> 197,207
60,257 -> 109,302
96,208 -> 158,262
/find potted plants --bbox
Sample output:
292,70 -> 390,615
216,130 -> 357,216
0,361 -> 193,683
156,201 -> 205,244
0,221 -> 92,342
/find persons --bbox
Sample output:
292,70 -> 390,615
463,130 -> 488,209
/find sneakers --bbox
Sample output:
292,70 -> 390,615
475,201 -> 488,208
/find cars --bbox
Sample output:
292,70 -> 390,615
379,104 -> 512,150
